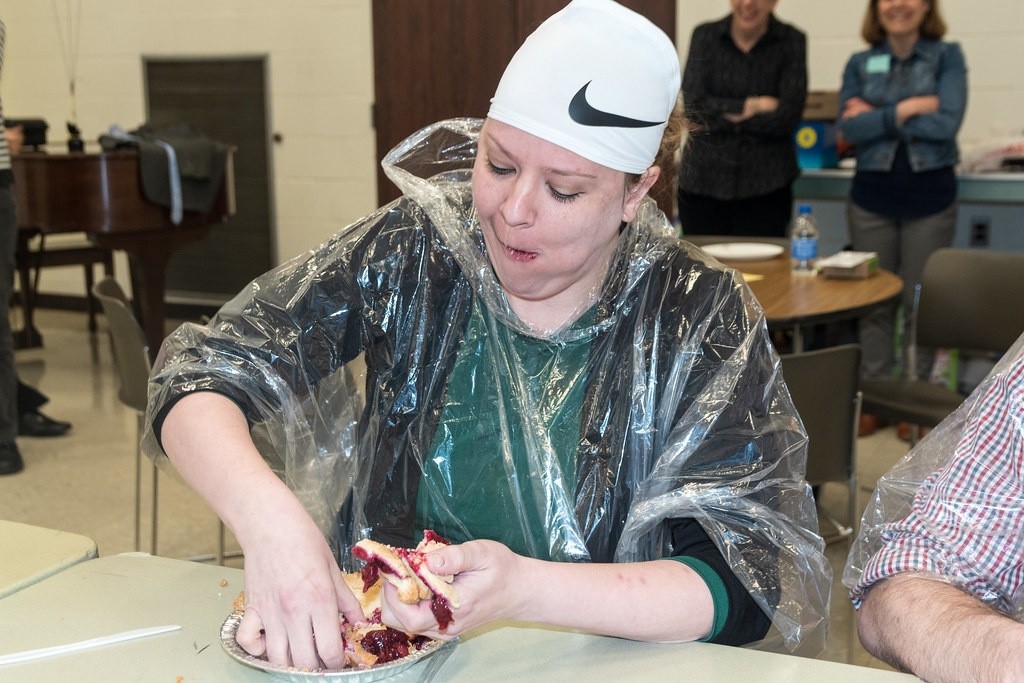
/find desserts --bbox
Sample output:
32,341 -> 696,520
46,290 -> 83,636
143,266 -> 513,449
221,530 -> 457,683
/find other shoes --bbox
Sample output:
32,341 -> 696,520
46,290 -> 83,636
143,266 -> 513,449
899,421 -> 925,440
0,439 -> 23,475
18,408 -> 71,434
858,404 -> 890,434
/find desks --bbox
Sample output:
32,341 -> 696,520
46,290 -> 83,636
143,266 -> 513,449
1,520 -> 923,682
718,248 -> 905,352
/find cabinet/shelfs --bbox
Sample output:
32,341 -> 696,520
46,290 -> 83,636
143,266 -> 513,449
793,168 -> 1022,367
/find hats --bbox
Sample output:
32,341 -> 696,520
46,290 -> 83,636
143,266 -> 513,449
487,0 -> 681,174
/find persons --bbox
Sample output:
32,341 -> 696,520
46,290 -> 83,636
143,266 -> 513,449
856,338 -> 1023,682
142,0 -> 836,671
673,1 -> 808,352
838,1 -> 967,381
0,20 -> 72,476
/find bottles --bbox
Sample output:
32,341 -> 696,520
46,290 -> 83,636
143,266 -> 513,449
790,204 -> 818,274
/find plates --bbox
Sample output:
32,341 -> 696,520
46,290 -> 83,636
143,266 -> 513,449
703,243 -> 783,261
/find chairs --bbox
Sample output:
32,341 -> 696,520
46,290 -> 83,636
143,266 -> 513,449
779,342 -> 866,552
862,248 -> 1024,502
93,277 -> 243,566
